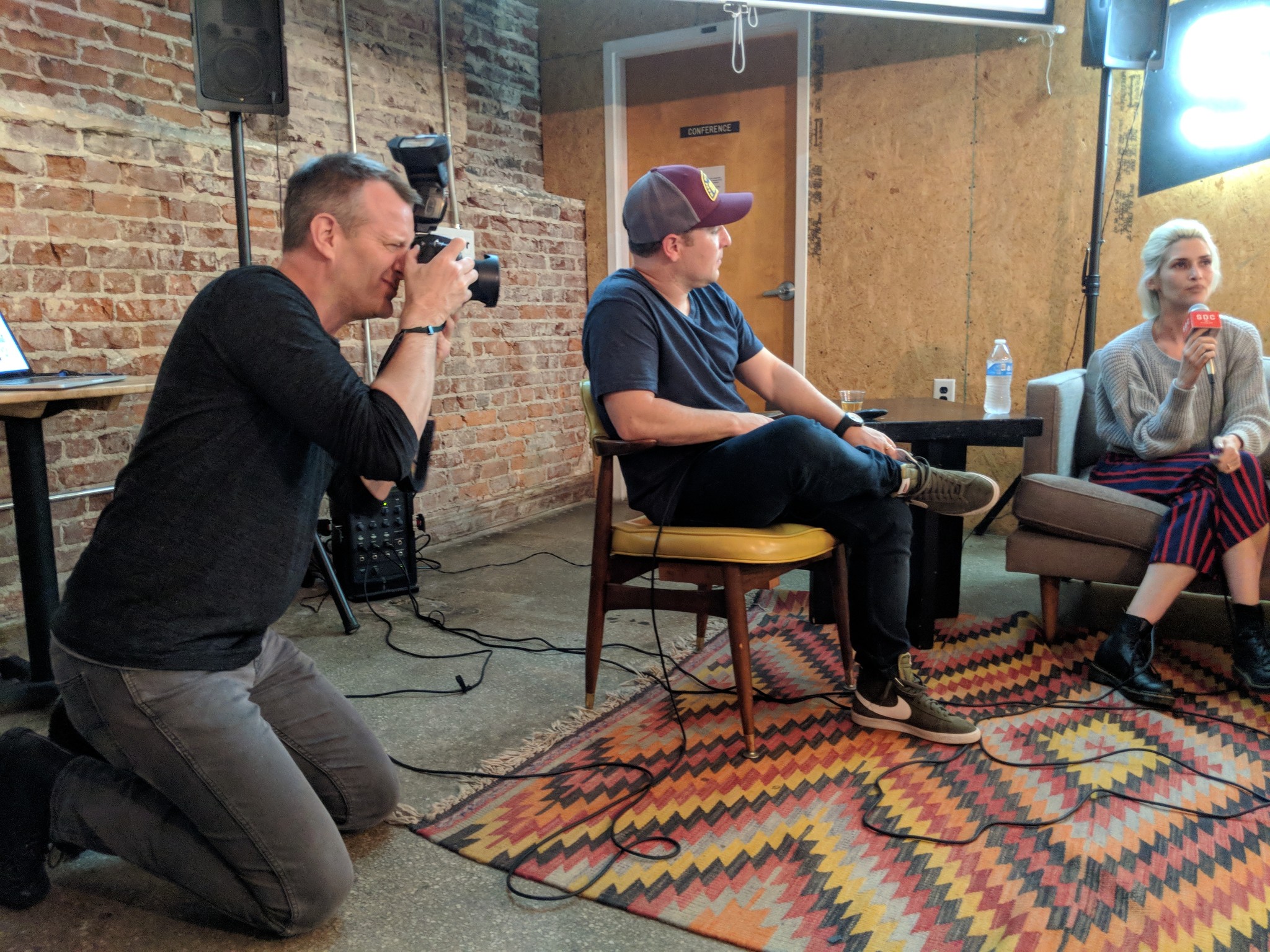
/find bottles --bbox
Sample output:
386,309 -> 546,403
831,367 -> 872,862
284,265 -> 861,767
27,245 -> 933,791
983,339 -> 1012,415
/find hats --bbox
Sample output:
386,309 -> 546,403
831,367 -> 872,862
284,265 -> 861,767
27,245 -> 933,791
621,165 -> 752,242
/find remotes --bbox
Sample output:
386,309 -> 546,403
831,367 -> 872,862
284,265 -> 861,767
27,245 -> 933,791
853,408 -> 888,419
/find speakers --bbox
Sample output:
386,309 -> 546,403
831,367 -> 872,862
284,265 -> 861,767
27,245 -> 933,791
189,0 -> 289,115
1081,0 -> 1170,70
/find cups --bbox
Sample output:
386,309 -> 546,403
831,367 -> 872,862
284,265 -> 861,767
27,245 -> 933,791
838,390 -> 867,413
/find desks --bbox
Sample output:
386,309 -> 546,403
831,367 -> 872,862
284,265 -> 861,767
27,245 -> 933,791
766,397 -> 1044,650
0,373 -> 159,712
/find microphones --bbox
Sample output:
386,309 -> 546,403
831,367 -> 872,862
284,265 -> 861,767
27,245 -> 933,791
1181,303 -> 1222,385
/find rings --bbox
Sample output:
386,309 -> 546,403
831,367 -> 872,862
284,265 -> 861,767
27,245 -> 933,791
1227,464 -> 1233,472
884,444 -> 893,452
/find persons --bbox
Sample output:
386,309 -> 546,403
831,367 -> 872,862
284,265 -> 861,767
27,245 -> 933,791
581,166 -> 1000,746
1,148 -> 479,939
1088,219 -> 1270,712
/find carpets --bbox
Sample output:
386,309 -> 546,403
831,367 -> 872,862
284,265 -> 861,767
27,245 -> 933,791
388,588 -> 1270,952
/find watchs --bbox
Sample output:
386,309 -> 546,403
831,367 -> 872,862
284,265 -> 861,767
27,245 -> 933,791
835,410 -> 863,441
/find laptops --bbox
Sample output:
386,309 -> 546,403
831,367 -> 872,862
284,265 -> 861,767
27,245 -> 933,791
0,311 -> 128,391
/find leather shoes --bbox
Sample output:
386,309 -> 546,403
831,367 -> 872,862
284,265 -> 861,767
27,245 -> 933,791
1086,604 -> 1177,707
1223,599 -> 1269,688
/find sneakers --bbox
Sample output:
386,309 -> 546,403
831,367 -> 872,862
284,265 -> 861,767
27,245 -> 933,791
49,696 -> 113,862
851,650 -> 982,745
893,451 -> 999,516
0,726 -> 75,911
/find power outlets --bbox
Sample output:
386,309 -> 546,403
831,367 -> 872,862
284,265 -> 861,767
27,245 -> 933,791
933,379 -> 955,402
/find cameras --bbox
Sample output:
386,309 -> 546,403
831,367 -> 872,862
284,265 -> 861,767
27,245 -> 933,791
387,134 -> 500,308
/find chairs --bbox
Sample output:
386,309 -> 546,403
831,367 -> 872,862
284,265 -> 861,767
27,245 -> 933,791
580,379 -> 856,759
1006,349 -> 1270,640
